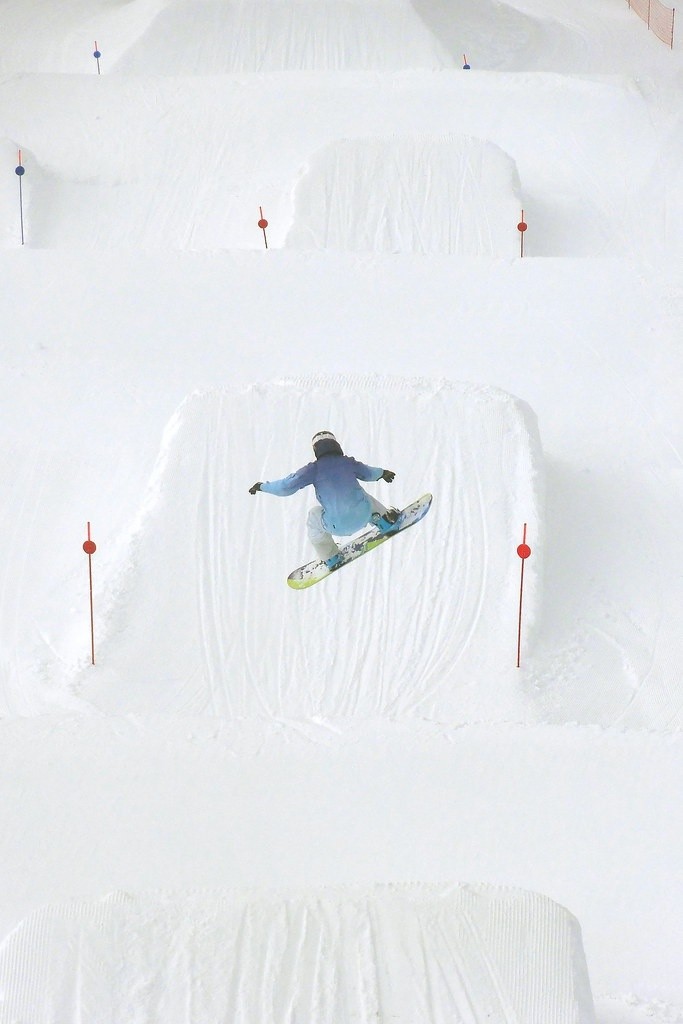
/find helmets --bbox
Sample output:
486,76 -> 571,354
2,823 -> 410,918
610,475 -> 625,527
312,431 -> 336,451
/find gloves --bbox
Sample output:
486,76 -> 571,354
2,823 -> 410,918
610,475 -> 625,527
249,482 -> 262,495
380,470 -> 395,483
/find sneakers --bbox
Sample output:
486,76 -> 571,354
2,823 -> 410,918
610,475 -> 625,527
377,506 -> 399,532
326,551 -> 345,571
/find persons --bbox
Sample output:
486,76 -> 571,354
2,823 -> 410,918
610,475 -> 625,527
247,431 -> 396,571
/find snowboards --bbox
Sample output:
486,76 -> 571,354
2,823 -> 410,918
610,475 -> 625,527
287,493 -> 433,590
